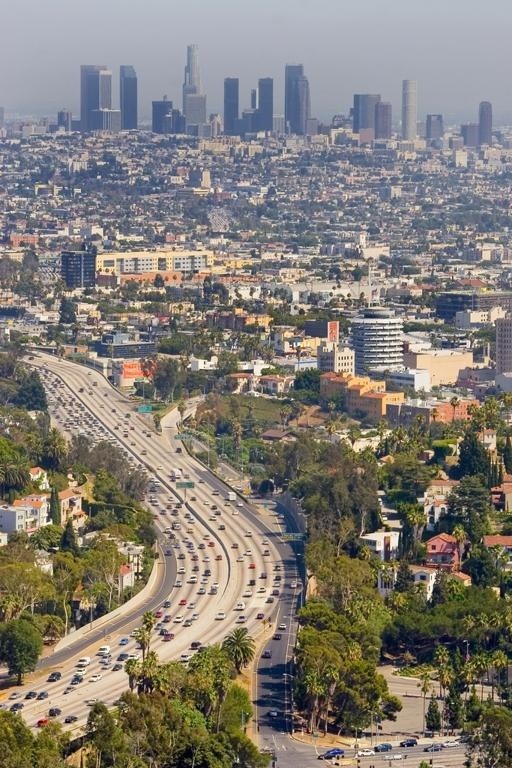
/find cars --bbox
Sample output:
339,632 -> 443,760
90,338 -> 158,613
317,736 -> 469,768
1,356 -> 297,729
